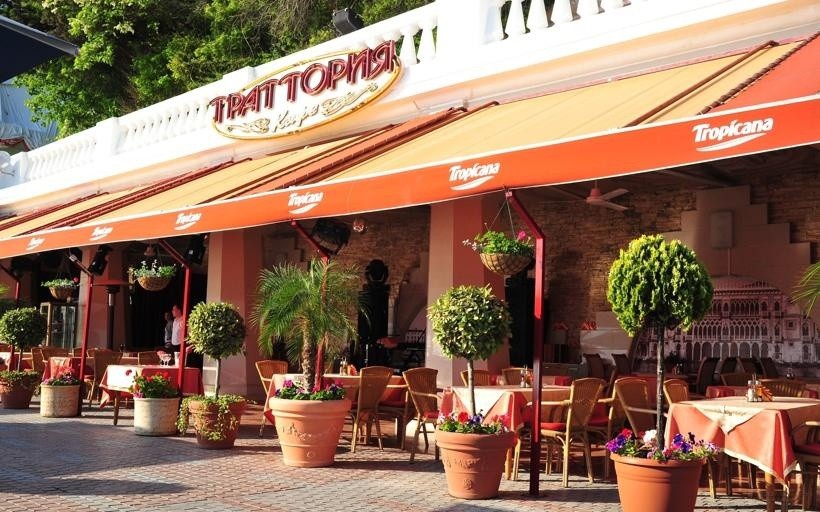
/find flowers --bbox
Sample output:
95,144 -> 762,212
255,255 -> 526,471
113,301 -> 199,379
42,369 -> 82,386
132,374 -> 178,400
608,428 -> 715,460
434,412 -> 506,437
272,379 -> 345,402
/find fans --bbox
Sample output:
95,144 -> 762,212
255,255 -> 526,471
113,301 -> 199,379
568,187 -> 631,212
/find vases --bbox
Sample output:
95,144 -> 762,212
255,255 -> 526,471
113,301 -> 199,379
609,450 -> 707,512
41,386 -> 79,418
269,401 -> 351,465
133,399 -> 180,436
433,433 -> 510,499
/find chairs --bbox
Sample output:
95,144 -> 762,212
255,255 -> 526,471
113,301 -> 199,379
662,379 -> 718,499
73,347 -> 85,357
512,377 -> 607,487
588,379 -> 626,481
255,360 -> 296,438
614,378 -> 672,445
31,347 -> 46,383
686,354 -> 720,393
83,350 -> 122,406
722,356 -> 737,388
41,346 -> 67,365
788,421 -> 820,511
138,350 -> 162,367
362,335 -> 394,366
367,382 -> 428,449
722,372 -> 749,385
740,356 -> 756,385
403,366 -> 447,463
502,367 -> 534,387
612,353 -> 640,375
460,369 -> 493,387
345,366 -> 389,452
762,376 -> 809,406
759,356 -> 779,378
583,354 -> 608,377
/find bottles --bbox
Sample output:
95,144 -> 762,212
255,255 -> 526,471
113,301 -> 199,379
520,369 -> 525,388
786,368 -> 794,378
679,363 -> 684,375
756,381 -> 763,402
748,380 -> 754,402
526,371 -> 531,388
340,360 -> 343,375
343,361 -> 347,375
675,363 -> 680,375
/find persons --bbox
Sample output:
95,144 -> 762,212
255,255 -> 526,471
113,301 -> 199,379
162,309 -> 175,365
170,302 -> 193,365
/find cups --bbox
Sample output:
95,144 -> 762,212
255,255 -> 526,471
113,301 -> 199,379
120,344 -> 126,353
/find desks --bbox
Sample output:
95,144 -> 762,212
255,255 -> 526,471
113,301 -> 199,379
398,342 -> 425,369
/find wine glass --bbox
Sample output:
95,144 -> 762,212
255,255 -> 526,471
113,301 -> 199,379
163,354 -> 168,367
167,355 -> 171,367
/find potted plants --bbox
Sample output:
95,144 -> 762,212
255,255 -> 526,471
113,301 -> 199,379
462,224 -> 535,276
132,260 -> 177,291
183,393 -> 247,449
41,278 -> 77,298
1,370 -> 36,408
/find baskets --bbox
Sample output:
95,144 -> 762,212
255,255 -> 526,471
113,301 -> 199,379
480,253 -> 531,275
50,288 -> 71,299
138,277 -> 169,291
